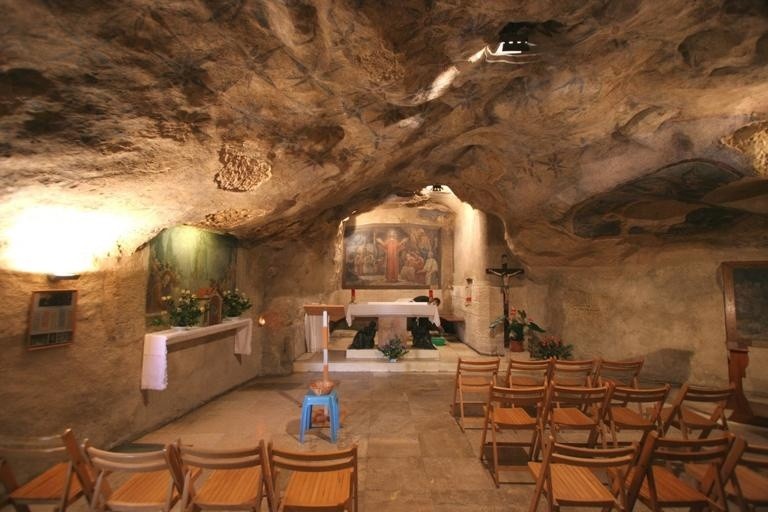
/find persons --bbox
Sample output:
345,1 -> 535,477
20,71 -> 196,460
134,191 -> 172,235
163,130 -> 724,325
409,296 -> 440,336
344,228 -> 441,285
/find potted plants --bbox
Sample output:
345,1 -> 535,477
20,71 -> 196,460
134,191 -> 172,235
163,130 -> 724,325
377,338 -> 409,362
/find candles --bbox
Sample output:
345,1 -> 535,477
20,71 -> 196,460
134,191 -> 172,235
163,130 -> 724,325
352,288 -> 355,296
429,290 -> 433,296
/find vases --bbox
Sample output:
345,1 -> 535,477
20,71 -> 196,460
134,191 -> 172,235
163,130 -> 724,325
511,337 -> 523,352
224,317 -> 240,323
172,326 -> 191,331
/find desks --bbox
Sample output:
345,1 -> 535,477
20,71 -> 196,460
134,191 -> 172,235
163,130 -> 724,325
346,302 -> 441,349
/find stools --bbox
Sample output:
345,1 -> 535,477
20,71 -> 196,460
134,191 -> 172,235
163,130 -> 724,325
300,391 -> 339,443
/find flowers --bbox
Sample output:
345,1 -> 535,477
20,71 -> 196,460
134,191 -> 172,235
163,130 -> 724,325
159,290 -> 205,326
531,336 -> 575,359
223,289 -> 253,316
489,307 -> 546,340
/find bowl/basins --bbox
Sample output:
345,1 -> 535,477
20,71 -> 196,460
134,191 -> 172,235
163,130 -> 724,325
310,381 -> 335,395
